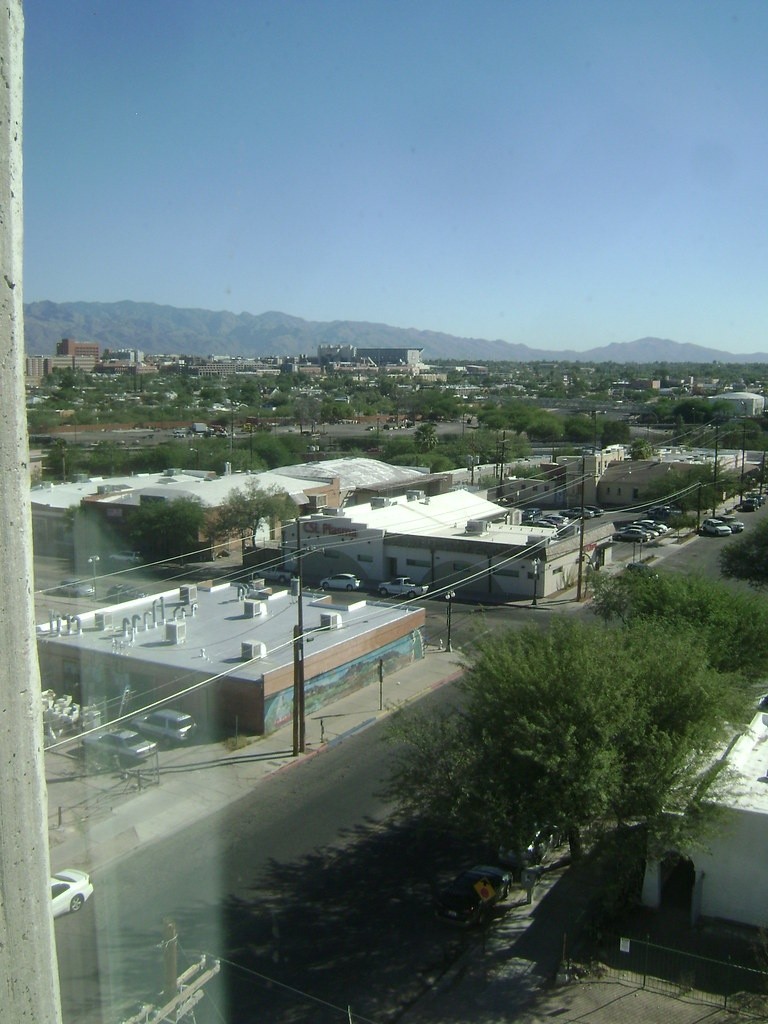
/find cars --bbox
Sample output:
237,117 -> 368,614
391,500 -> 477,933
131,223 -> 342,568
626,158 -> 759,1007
433,864 -> 514,930
57,577 -> 96,597
49,868 -> 95,919
78,726 -> 156,760
622,525 -> 660,538
752,494 -> 766,504
612,528 -> 653,543
517,503 -> 604,537
632,519 -> 669,534
743,498 -> 760,511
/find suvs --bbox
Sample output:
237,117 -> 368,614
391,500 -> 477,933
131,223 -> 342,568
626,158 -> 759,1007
109,550 -> 145,564
702,518 -> 732,537
319,573 -> 362,592
132,709 -> 197,743
719,514 -> 745,533
107,583 -> 146,603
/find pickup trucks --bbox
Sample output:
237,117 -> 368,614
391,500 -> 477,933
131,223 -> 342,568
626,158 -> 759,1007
377,575 -> 429,599
252,563 -> 295,583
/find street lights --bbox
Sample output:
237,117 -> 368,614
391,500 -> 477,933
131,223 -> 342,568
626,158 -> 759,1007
87,554 -> 101,587
